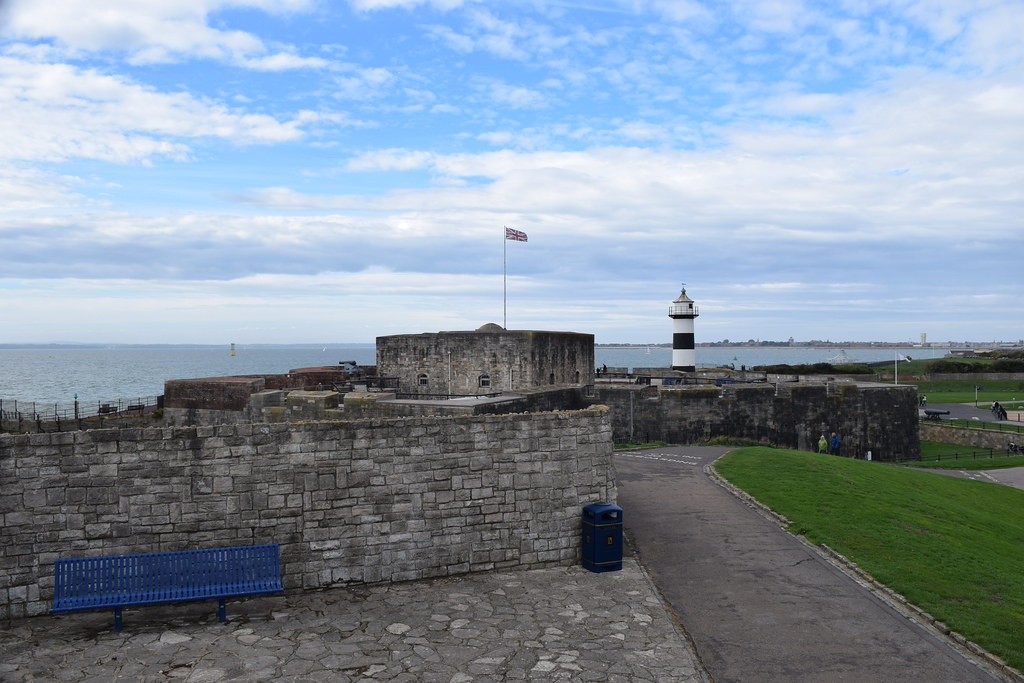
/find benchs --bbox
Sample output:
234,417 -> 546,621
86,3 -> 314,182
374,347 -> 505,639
49,544 -> 283,632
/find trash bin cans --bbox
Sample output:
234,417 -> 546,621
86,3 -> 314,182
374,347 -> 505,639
581,501 -> 624,573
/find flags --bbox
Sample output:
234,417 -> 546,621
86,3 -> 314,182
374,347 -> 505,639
506,227 -> 528,242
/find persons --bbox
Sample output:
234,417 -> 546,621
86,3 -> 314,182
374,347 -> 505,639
919,394 -> 927,406
830,433 -> 839,455
596,368 -> 600,378
1008,440 -> 1016,453
603,364 -> 607,373
992,400 -> 999,413
818,436 -> 828,454
